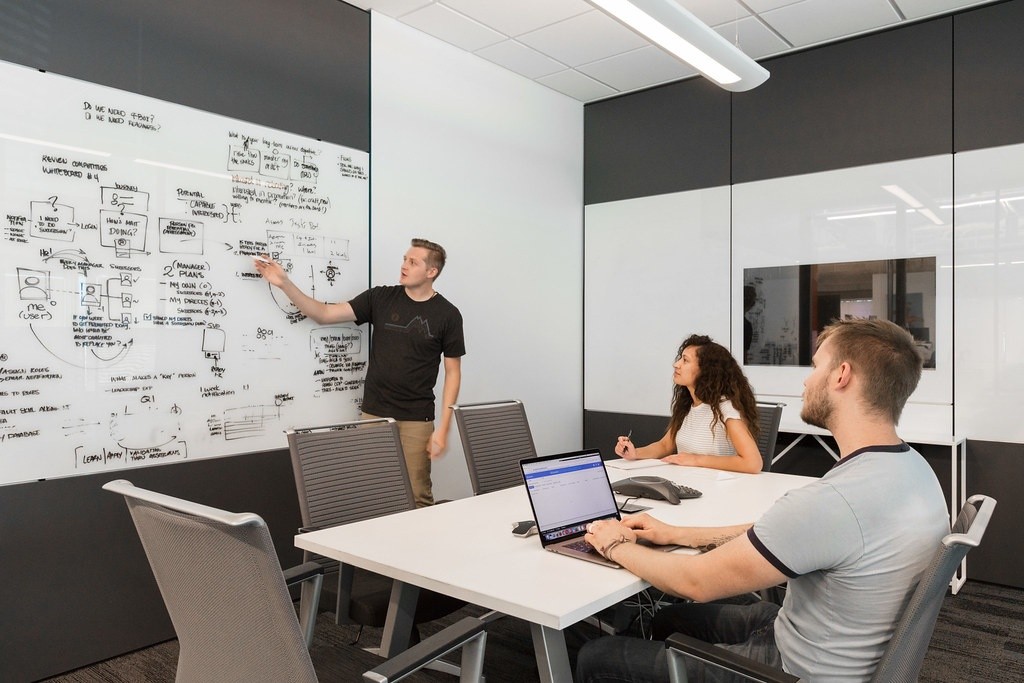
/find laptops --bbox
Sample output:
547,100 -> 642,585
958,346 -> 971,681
517,447 -> 682,568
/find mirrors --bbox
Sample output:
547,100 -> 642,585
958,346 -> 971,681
744,257 -> 937,371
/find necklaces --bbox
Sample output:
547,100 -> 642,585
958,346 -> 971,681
431,291 -> 435,298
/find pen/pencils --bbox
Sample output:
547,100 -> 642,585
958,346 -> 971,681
622,426 -> 635,454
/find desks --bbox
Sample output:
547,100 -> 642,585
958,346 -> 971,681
294,458 -> 823,683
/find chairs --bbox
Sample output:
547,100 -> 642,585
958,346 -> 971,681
282,418 -> 469,650
454,401 -> 538,495
749,401 -> 783,472
664,495 -> 999,683
100,479 -> 490,683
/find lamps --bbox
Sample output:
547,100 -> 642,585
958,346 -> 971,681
584,0 -> 770,93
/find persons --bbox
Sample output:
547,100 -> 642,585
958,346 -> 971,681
252,239 -> 467,509
572,315 -> 950,683
615,334 -> 762,475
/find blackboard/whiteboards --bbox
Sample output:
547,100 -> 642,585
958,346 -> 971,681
744,265 -> 800,367
0,61 -> 375,489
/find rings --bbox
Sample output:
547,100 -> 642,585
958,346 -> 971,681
587,523 -> 596,533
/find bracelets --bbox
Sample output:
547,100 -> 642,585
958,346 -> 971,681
601,534 -> 634,562
